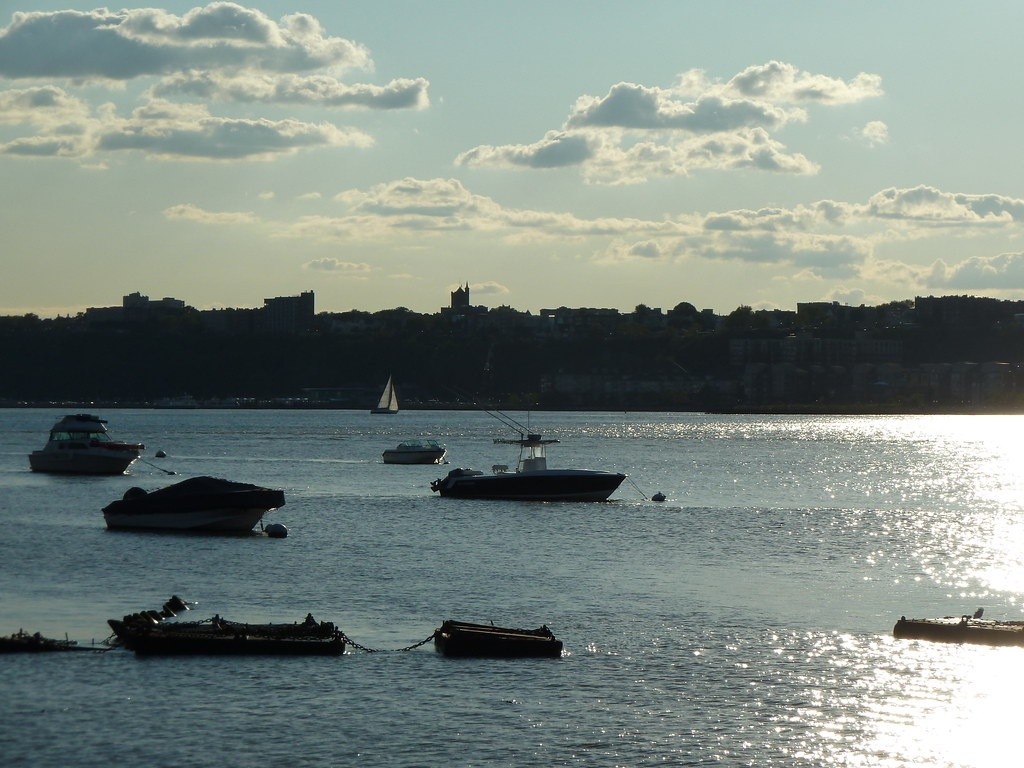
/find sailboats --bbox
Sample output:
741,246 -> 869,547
370,374 -> 399,413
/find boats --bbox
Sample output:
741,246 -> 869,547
895,613 -> 1024,649
106,613 -> 345,654
383,439 -> 445,464
27,413 -> 145,478
101,478 -> 279,534
430,380 -> 627,501
433,619 -> 563,658
